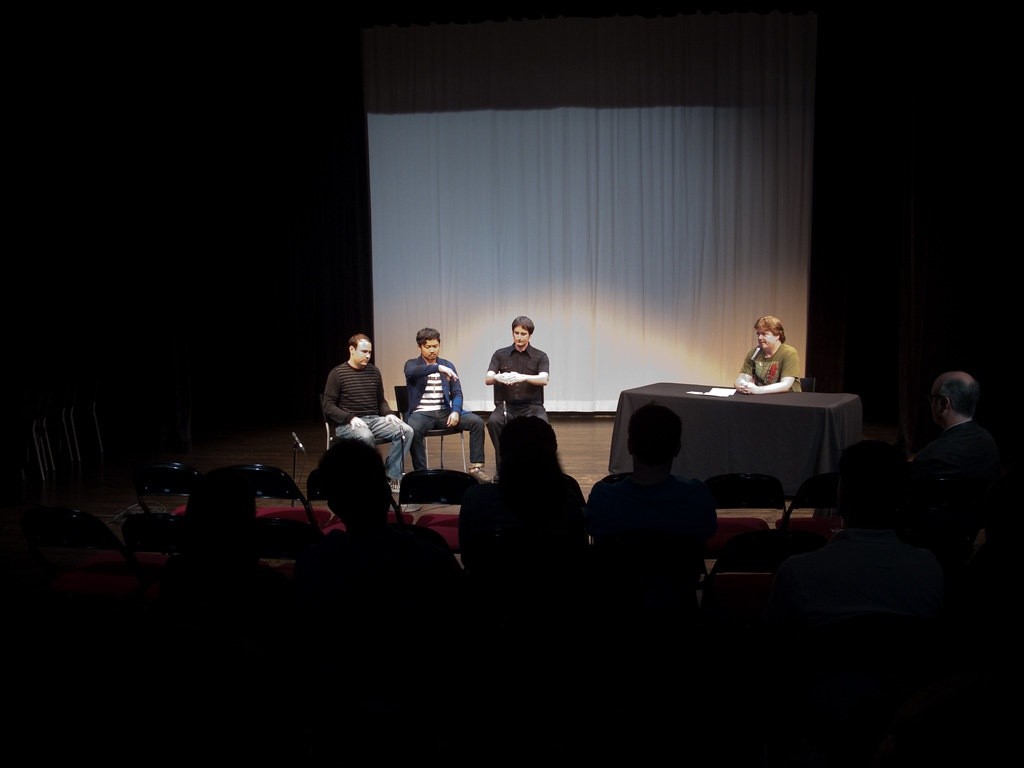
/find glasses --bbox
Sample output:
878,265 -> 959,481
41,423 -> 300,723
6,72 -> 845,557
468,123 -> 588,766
927,396 -> 941,404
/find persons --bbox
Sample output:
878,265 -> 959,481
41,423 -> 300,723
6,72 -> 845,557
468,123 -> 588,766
15,468 -> 139,566
485,317 -> 550,477
292,371 -> 1000,618
405,330 -> 492,484
738,316 -> 802,396
323,333 -> 416,515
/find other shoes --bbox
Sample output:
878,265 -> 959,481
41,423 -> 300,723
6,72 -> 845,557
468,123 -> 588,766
469,466 -> 492,481
387,479 -> 400,493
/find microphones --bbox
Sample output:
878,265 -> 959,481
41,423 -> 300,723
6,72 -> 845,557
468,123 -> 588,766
400,426 -> 406,443
751,346 -> 762,361
292,432 -> 306,453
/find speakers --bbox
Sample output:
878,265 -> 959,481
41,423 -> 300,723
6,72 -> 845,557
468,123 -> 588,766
143,450 -> 198,496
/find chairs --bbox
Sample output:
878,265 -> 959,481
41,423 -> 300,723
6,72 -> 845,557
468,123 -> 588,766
1,463 -> 1024,768
319,394 -> 393,450
394,386 -> 466,472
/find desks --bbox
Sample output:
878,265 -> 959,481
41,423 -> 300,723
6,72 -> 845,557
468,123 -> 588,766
608,382 -> 864,496
